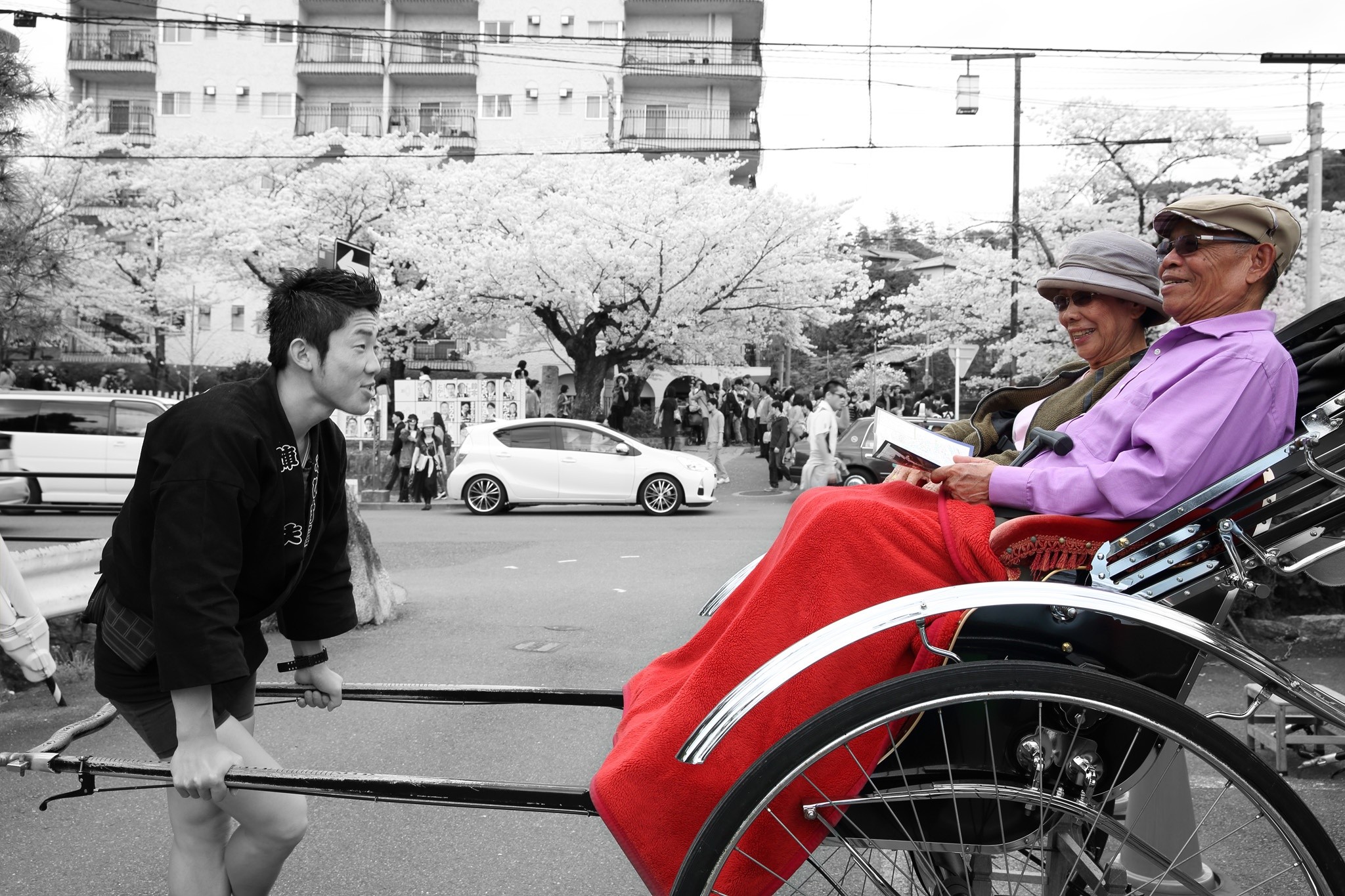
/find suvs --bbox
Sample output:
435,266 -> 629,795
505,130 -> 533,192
0,389 -> 186,515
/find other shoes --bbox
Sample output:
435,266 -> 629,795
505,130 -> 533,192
421,504 -> 431,510
434,491 -> 448,500
756,454 -> 763,458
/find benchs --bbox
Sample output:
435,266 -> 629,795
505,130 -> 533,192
910,466 -> 1276,655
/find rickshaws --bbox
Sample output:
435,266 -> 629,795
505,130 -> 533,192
0,295 -> 1344,894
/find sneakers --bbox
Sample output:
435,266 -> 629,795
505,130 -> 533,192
765,486 -> 779,492
717,476 -> 730,484
789,482 -> 798,490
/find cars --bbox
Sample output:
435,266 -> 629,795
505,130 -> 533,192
444,419 -> 718,513
782,414 -> 967,491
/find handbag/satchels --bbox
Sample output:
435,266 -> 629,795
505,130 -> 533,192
688,414 -> 703,425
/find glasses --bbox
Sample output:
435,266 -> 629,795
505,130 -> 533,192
1155,233 -> 1260,255
831,392 -> 846,399
1051,292 -> 1107,312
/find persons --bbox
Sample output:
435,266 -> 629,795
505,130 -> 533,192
456,382 -> 469,398
590,372 -> 954,491
695,229 -> 1168,618
556,385 -> 572,419
416,380 -> 433,401
509,402 -> 517,417
483,402 -> 496,422
589,191 -> 1297,834
346,418 -> 374,438
483,381 -> 497,401
510,360 -> 542,418
374,375 -> 393,416
385,411 -> 449,510
439,402 -> 451,422
445,383 -> 455,399
503,380 -> 514,401
461,401 -> 474,422
78,265 -> 385,896
544,414 -> 556,418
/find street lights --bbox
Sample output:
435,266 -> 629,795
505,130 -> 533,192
951,51 -> 1036,340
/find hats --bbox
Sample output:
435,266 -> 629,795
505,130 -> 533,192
614,372 -> 629,386
1036,230 -> 1171,326
28,368 -> 39,373
1152,194 -> 1302,280
420,420 -> 436,429
